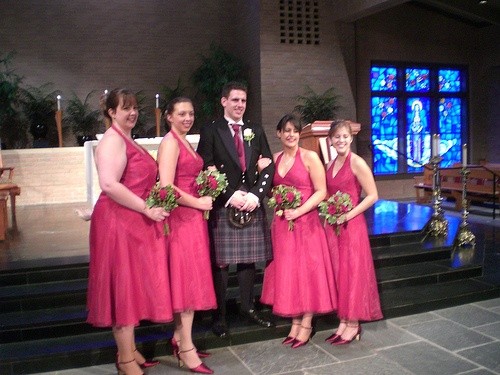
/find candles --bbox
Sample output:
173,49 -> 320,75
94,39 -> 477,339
105,90 -> 108,99
432,134 -> 441,156
155,94 -> 159,108
462,144 -> 468,167
56,95 -> 61,111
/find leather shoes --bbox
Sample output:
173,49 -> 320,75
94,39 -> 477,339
212,321 -> 229,338
239,308 -> 276,328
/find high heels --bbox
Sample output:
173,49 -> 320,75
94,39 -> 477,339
282,323 -> 301,344
177,345 -> 214,374
331,323 -> 361,345
291,326 -> 312,349
172,337 -> 211,359
325,322 -> 346,342
133,348 -> 155,368
116,352 -> 148,375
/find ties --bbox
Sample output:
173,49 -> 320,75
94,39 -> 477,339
231,124 -> 246,173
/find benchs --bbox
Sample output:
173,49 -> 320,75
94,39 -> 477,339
415,169 -> 500,209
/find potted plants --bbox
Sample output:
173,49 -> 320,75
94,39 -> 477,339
23,84 -> 148,150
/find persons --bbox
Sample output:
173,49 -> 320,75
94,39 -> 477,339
255,114 -> 339,348
155,96 -> 217,373
321,120 -> 384,345
84,87 -> 174,375
196,80 -> 274,338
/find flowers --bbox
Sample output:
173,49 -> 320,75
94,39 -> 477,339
244,128 -> 255,147
145,181 -> 181,236
267,185 -> 304,231
196,169 -> 230,220
318,191 -> 355,236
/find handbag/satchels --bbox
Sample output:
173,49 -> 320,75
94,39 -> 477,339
229,205 -> 253,227
